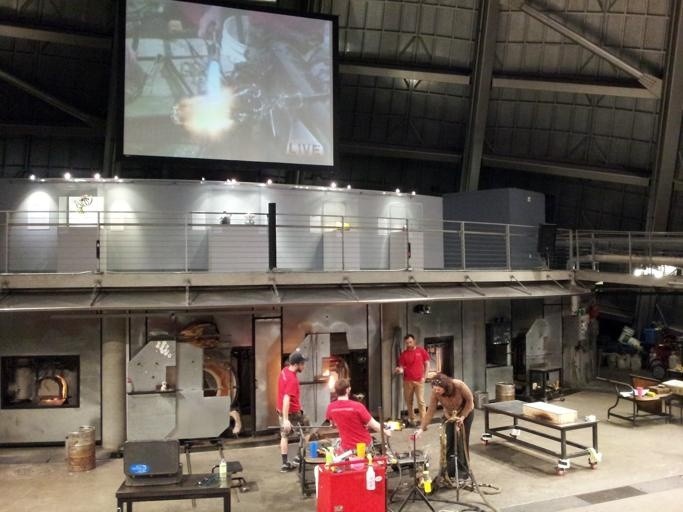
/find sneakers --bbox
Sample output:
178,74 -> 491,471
281,463 -> 296,472
411,420 -> 417,426
293,454 -> 304,463
444,476 -> 471,488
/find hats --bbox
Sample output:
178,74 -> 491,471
289,352 -> 308,364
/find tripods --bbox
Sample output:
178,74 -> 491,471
435,409 -> 474,502
398,437 -> 436,512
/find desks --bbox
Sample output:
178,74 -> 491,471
485,400 -> 599,473
531,366 -> 564,399
114,475 -> 232,512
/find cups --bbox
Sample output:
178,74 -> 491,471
308,439 -> 318,458
356,443 -> 366,459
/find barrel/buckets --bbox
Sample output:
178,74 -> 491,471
628,337 -> 640,347
495,382 -> 515,400
618,325 -> 635,344
650,357 -> 665,378
65,425 -> 96,472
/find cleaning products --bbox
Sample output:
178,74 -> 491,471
366,453 -> 376,491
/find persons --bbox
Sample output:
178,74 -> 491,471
275,351 -> 310,473
413,373 -> 474,480
395,334 -> 431,431
327,379 -> 391,454
